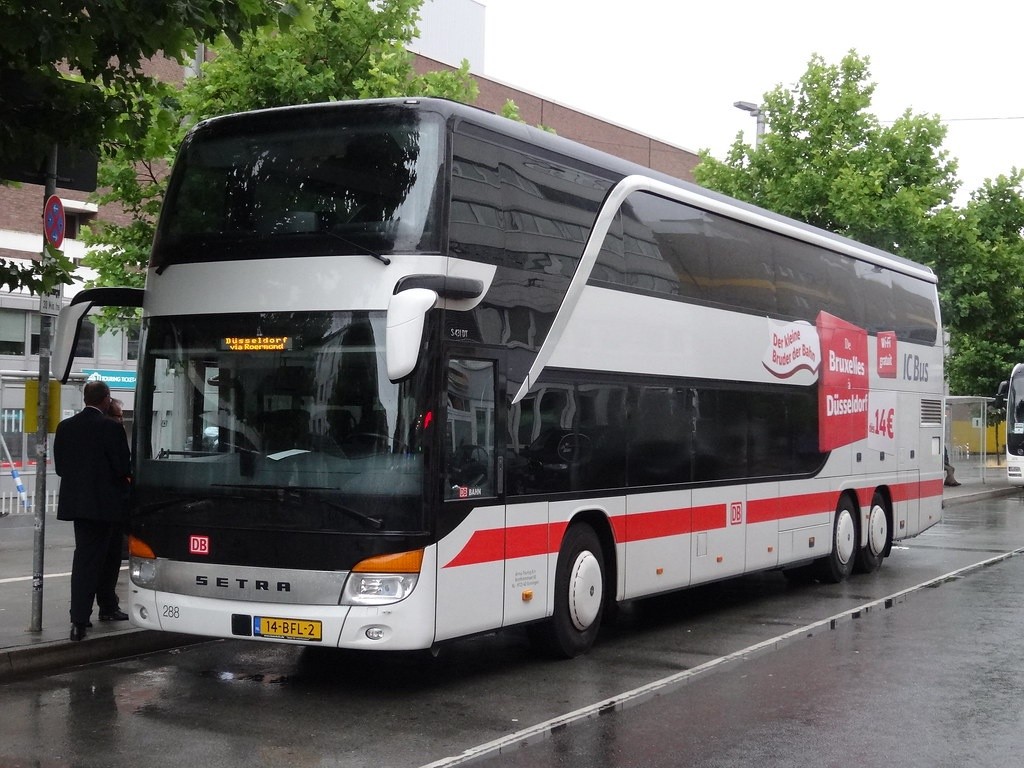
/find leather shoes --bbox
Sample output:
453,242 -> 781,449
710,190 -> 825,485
70,622 -> 86,641
87,620 -> 93,628
99,609 -> 129,621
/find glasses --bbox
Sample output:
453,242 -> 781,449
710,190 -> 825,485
111,412 -> 124,421
108,397 -> 113,404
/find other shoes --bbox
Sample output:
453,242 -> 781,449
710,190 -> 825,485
944,479 -> 961,486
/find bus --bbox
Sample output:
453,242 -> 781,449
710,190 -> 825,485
49,96 -> 944,651
995,362 -> 1024,489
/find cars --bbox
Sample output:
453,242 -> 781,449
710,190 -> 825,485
183,427 -> 219,457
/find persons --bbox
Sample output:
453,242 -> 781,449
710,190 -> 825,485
944,447 -> 961,486
53,381 -> 132,641
